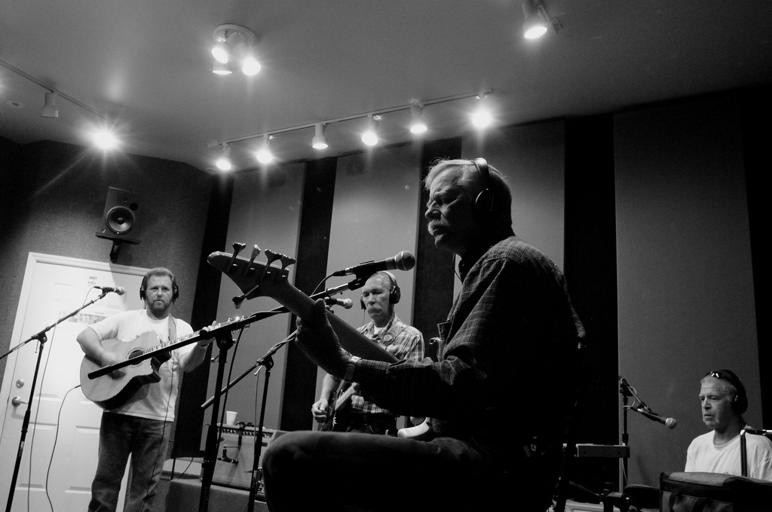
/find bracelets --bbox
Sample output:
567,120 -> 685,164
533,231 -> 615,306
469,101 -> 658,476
345,355 -> 361,382
199,341 -> 210,348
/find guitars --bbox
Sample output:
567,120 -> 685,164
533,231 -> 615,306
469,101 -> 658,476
316,380 -> 362,433
79,314 -> 249,411
205,242 -> 438,438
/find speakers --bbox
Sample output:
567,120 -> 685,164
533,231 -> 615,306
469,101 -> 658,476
95,186 -> 144,244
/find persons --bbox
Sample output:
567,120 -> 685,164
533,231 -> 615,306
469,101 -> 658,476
259,157 -> 586,512
684,366 -> 772,482
76,267 -> 216,512
311,269 -> 425,439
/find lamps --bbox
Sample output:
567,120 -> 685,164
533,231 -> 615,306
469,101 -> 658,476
520,0 -> 556,42
39,58 -> 59,120
209,23 -> 264,76
206,88 -> 496,172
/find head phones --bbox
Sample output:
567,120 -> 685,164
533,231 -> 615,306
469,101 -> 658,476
472,157 -> 503,226
140,267 -> 179,302
706,370 -> 748,416
360,271 -> 400,309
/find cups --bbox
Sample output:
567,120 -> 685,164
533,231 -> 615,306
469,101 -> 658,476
227,411 -> 238,427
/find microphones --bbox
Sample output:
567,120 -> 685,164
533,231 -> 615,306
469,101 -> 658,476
332,251 -> 416,277
94,286 -> 125,295
637,408 -> 676,430
325,298 -> 352,310
